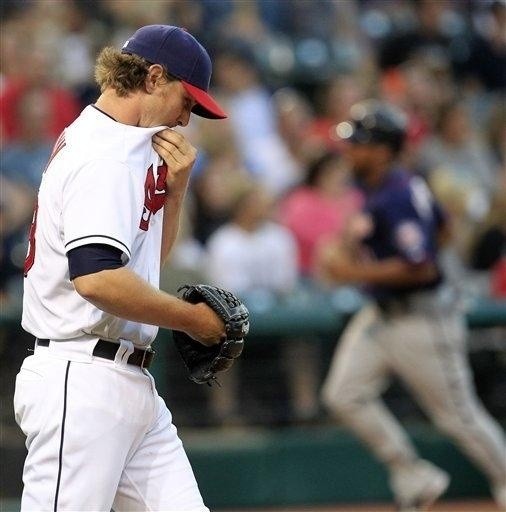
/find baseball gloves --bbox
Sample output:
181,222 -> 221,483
172,284 -> 250,387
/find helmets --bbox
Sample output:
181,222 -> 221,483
332,102 -> 408,164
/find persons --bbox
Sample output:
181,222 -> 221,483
0,0 -> 505,425
317,98 -> 505,512
10,22 -> 229,511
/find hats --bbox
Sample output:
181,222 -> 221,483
121,24 -> 229,120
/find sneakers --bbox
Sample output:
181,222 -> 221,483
385,457 -> 456,512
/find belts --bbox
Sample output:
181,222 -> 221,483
357,275 -> 450,316
35,334 -> 156,370
